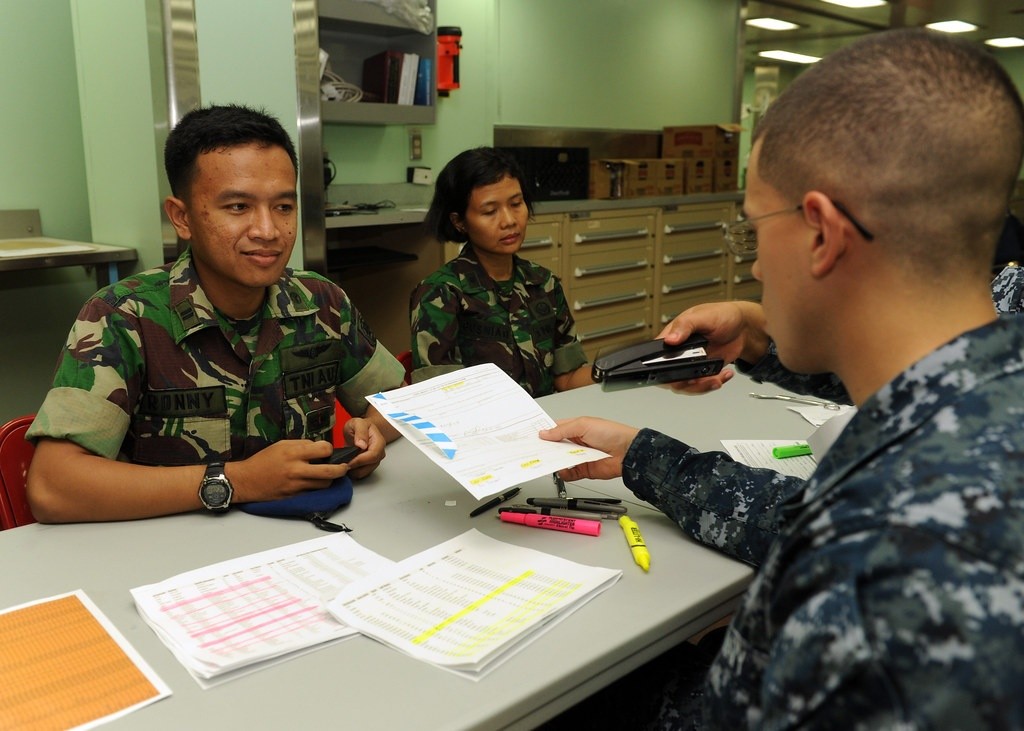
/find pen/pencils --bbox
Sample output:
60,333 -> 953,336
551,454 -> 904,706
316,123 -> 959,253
470,486 -> 522,518
750,391 -> 824,409
498,471 -> 627,519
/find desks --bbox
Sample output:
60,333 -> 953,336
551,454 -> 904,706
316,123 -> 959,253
1,237 -> 138,290
1,363 -> 855,731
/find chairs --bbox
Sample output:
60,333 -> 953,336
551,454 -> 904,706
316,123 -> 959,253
2,415 -> 44,530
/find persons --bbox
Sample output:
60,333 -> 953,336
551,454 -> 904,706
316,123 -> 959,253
409,147 -> 599,398
539,30 -> 1024,731
26,106 -> 408,526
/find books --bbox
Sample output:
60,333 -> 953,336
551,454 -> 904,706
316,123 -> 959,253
361,48 -> 431,107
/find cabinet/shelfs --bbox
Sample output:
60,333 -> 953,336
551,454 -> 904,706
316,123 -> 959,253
316,0 -> 437,126
515,187 -> 767,368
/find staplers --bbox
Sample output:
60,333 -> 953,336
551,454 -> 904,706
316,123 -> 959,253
590,337 -> 727,391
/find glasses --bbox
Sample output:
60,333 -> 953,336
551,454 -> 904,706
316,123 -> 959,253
721,199 -> 874,256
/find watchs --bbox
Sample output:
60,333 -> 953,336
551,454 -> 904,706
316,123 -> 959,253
197,461 -> 235,516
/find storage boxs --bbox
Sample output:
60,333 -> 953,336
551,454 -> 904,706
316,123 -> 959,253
589,124 -> 743,199
495,145 -> 590,202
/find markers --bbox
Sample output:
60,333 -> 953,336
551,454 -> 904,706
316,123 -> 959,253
618,515 -> 654,574
770,443 -> 812,459
499,512 -> 602,536
